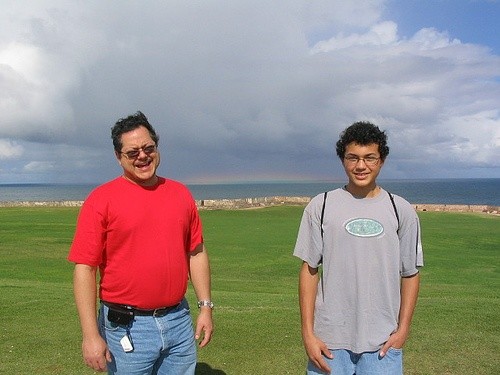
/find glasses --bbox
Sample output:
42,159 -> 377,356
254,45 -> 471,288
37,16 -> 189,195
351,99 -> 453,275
119,145 -> 156,158
343,156 -> 381,165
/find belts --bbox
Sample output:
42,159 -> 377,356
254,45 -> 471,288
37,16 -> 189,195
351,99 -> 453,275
100,300 -> 181,318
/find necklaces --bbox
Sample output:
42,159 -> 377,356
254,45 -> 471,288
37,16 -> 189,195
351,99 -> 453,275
344,184 -> 361,198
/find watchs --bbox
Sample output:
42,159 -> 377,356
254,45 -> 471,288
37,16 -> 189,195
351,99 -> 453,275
196,300 -> 214,310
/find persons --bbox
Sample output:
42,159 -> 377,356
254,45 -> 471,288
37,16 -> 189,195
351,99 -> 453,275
67,110 -> 215,375
292,122 -> 425,375
